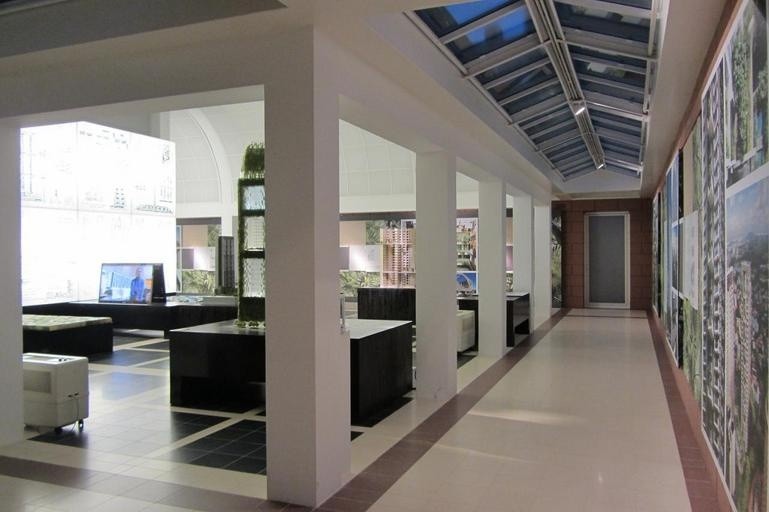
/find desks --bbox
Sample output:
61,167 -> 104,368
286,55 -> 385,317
357,288 -> 530,347
19,294 -> 237,364
164,294 -> 412,423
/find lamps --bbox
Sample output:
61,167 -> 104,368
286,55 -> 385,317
572,99 -> 586,115
594,155 -> 604,169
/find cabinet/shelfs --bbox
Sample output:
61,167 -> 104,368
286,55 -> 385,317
380,226 -> 416,288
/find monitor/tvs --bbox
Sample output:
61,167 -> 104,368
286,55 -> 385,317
152,264 -> 166,302
457,271 -> 478,296
98,263 -> 155,305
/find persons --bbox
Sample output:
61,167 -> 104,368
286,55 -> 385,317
129,266 -> 146,305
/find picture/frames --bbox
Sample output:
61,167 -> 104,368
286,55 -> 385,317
651,148 -> 684,370
